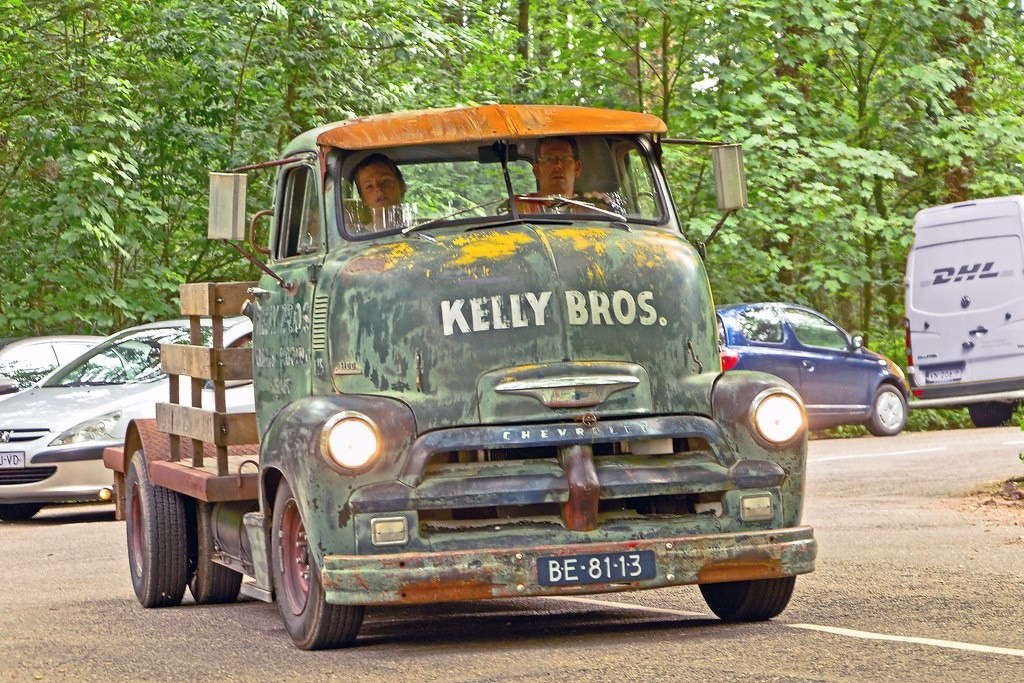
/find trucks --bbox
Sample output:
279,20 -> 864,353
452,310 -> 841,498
102,103 -> 818,651
903,194 -> 1024,428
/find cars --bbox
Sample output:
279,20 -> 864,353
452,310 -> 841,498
714,301 -> 911,438
0,334 -> 152,403
0,318 -> 255,523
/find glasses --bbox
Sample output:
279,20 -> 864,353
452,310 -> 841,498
535,156 -> 569,163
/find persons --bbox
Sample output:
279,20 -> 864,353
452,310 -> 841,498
497,136 -> 612,215
355,152 -> 433,232
